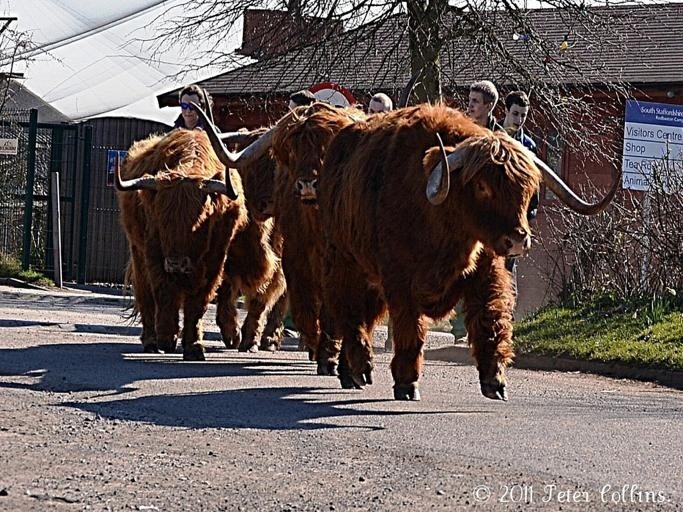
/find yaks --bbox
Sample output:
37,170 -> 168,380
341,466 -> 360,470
112,98 -> 626,403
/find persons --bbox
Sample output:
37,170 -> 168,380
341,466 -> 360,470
286,88 -> 315,109
467,76 -> 509,142
367,92 -> 394,114
171,80 -> 212,130
498,85 -> 539,222
